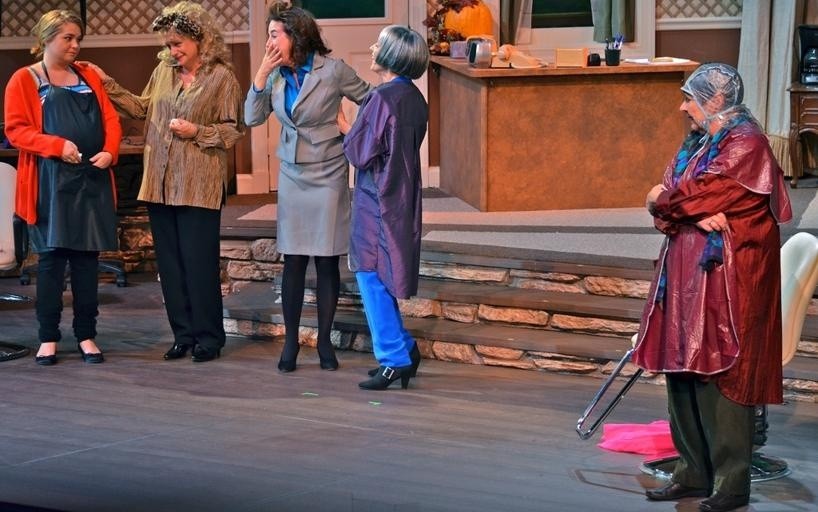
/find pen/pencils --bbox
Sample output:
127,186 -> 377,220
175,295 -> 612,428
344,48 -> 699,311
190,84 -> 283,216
606,32 -> 625,50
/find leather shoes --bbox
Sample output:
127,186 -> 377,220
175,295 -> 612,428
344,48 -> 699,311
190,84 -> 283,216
164,344 -> 224,362
78,344 -> 104,364
278,341 -> 339,372
35,350 -> 56,365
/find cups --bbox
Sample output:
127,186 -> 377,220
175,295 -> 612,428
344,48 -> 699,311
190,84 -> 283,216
605,47 -> 621,66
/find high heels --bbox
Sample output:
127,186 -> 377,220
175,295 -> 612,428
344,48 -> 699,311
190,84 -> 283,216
359,339 -> 421,390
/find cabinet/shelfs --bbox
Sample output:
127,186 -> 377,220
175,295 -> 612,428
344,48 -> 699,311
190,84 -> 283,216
0,30 -> 249,157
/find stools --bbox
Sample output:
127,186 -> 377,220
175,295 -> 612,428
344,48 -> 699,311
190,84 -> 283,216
13,212 -> 129,288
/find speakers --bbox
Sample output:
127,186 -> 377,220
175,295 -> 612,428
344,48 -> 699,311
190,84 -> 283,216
797,24 -> 818,86
466,40 -> 493,69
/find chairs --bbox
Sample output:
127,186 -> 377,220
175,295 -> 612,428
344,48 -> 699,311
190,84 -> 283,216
641,231 -> 818,483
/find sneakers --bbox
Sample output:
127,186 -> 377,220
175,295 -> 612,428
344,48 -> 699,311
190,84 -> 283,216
645,483 -> 750,512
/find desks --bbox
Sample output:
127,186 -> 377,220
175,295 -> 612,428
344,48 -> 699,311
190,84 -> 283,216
786,81 -> 817,188
427,54 -> 701,211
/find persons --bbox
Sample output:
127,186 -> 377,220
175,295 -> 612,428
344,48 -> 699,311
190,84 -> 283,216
74,1 -> 247,363
340,23 -> 430,391
243,0 -> 376,374
2,8 -> 123,366
632,62 -> 793,512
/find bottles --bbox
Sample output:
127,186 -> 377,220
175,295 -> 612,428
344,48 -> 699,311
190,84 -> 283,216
804,49 -> 818,72
